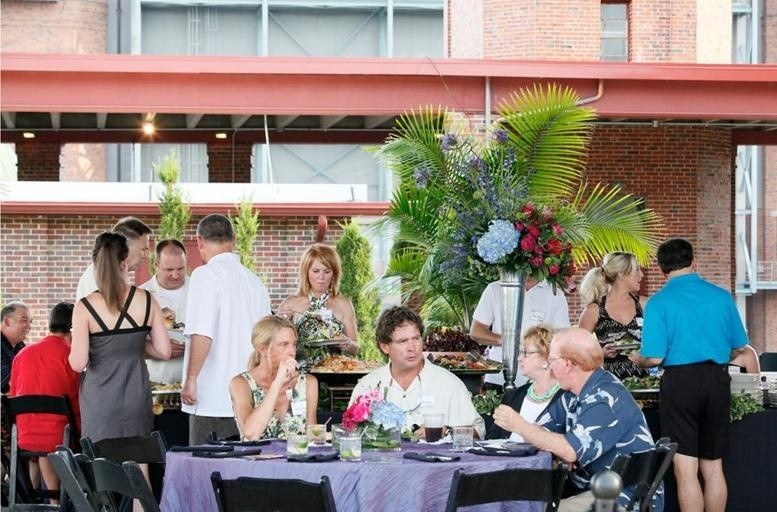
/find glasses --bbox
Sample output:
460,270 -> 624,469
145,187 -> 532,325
521,348 -> 543,358
383,373 -> 423,415
546,356 -> 563,364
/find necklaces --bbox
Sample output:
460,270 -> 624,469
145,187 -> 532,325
527,383 -> 560,403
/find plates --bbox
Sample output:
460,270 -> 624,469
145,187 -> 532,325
451,370 -> 501,374
308,339 -> 348,346
151,390 -> 181,394
609,344 -> 638,350
631,389 -> 660,392
729,373 -> 764,404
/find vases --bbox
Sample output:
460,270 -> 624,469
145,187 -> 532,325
498,264 -> 524,394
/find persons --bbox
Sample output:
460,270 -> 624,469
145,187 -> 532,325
10,302 -> 82,506
68,232 -> 171,512
76,217 -> 152,304
136,239 -> 191,410
277,244 -> 360,356
229,315 -> 318,441
493,328 -> 665,512
469,276 -> 570,434
484,323 -> 565,441
628,239 -> 750,511
728,344 -> 761,376
347,306 -> 486,440
180,214 -> 271,446
577,252 -> 650,380
0,303 -> 33,483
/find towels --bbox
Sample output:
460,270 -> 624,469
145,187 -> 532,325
465,446 -> 540,457
207,439 -> 272,447
190,449 -> 263,458
170,443 -> 235,452
401,451 -> 460,464
287,452 -> 342,463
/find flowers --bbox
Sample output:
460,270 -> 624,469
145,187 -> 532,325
412,128 -> 573,295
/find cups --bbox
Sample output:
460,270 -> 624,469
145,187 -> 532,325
332,425 -> 346,448
287,435 -> 309,461
306,424 -> 326,445
453,426 -> 473,451
423,414 -> 444,443
340,433 -> 361,461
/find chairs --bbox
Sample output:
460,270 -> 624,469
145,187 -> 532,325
210,471 -> 336,512
560,443 -> 680,512
72,453 -> 162,512
80,432 -> 171,512
2,392 -> 81,512
444,461 -> 568,512
50,444 -> 102,512
